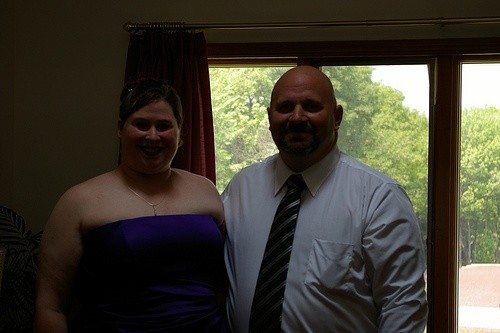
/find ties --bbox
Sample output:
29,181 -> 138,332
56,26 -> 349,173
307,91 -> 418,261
247,176 -> 307,333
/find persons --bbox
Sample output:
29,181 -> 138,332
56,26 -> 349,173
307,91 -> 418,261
216,65 -> 430,333
35,77 -> 232,332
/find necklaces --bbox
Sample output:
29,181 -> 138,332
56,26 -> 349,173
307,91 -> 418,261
115,167 -> 174,217
